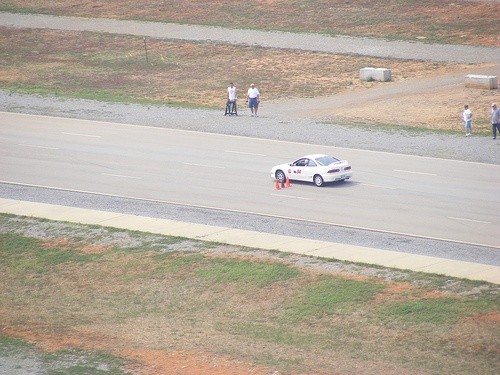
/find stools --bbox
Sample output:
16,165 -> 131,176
225,99 -> 237,115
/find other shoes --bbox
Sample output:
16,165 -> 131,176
493,137 -> 496,139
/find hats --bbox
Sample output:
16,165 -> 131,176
250,84 -> 255,86
491,103 -> 496,107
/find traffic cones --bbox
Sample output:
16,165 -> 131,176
281,179 -> 284,188
286,178 -> 290,187
276,180 -> 279,189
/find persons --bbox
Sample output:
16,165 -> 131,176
463,104 -> 472,136
227,83 -> 237,116
490,103 -> 500,139
246,83 -> 260,117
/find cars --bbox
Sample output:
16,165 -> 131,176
270,153 -> 352,188
